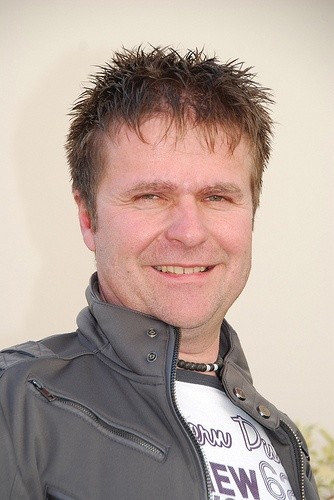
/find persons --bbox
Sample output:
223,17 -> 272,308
0,43 -> 325,499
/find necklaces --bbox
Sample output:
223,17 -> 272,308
106,310 -> 229,374
